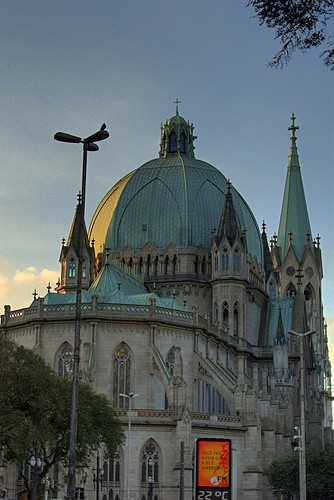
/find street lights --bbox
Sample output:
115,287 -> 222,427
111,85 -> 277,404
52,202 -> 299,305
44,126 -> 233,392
287,328 -> 316,500
52,122 -> 110,500
118,391 -> 139,500
29,456 -> 42,500
